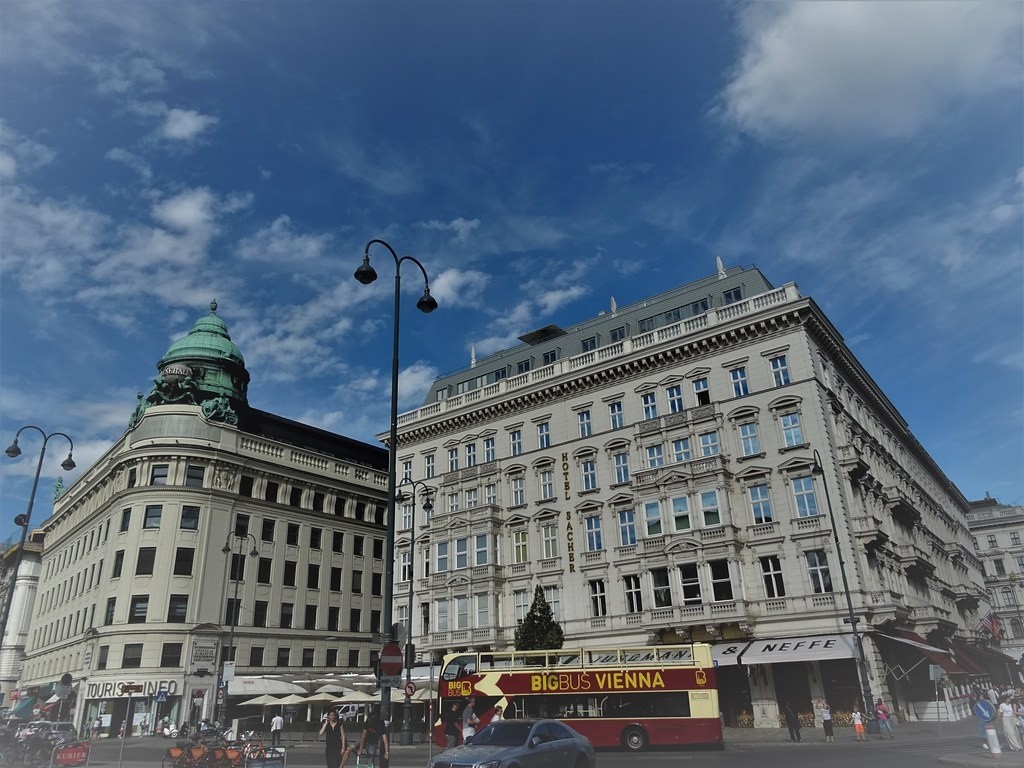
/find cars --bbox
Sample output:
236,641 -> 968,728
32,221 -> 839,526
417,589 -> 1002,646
19,721 -> 47,743
429,718 -> 597,768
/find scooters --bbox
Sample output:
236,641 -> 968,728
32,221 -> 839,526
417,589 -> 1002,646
177,722 -> 189,739
163,720 -> 178,739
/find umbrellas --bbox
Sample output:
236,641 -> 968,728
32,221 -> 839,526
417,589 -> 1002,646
238,688 -> 438,723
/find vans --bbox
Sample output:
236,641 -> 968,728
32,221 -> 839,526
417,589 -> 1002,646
322,703 -> 371,723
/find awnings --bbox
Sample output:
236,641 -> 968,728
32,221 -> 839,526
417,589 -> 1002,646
10,695 -> 36,720
741,633 -> 864,665
45,682 -> 78,703
877,628 -> 1014,677
370,641 -> 751,683
227,674 -> 374,695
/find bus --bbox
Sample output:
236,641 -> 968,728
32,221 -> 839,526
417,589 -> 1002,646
434,641 -> 724,752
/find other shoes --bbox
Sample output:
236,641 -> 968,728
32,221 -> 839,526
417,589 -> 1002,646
1000,745 -> 1004,748
983,743 -> 989,750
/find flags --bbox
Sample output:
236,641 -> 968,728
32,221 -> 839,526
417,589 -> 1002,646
981,610 -> 1002,643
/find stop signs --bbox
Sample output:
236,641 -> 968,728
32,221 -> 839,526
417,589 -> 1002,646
380,642 -> 403,676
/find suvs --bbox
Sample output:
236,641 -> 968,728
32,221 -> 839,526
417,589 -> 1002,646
39,722 -> 77,744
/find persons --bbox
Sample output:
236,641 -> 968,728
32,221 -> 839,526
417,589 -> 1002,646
462,697 -> 481,741
874,698 -> 894,739
214,466 -> 236,490
118,719 -> 125,739
491,706 -> 503,722
815,698 -> 835,743
319,710 -> 346,768
784,699 -> 802,743
140,715 -> 147,738
163,713 -> 169,728
340,713 -> 389,768
85,715 -> 103,740
181,717 -> 221,739
271,713 -> 284,745
850,706 -> 867,741
976,684 -> 1024,752
444,702 -> 462,749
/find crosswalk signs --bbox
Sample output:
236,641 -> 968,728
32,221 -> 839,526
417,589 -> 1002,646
155,691 -> 168,702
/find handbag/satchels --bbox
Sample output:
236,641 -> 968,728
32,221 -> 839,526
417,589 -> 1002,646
796,721 -> 801,729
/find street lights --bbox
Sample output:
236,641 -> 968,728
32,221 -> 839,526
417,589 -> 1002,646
394,479 -> 431,746
1010,575 -> 1024,630
353,238 -> 437,768
0,425 -> 76,647
220,529 -> 260,738
810,450 -> 880,735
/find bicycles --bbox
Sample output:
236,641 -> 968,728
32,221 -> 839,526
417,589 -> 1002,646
0,728 -> 86,768
161,724 -> 266,768
